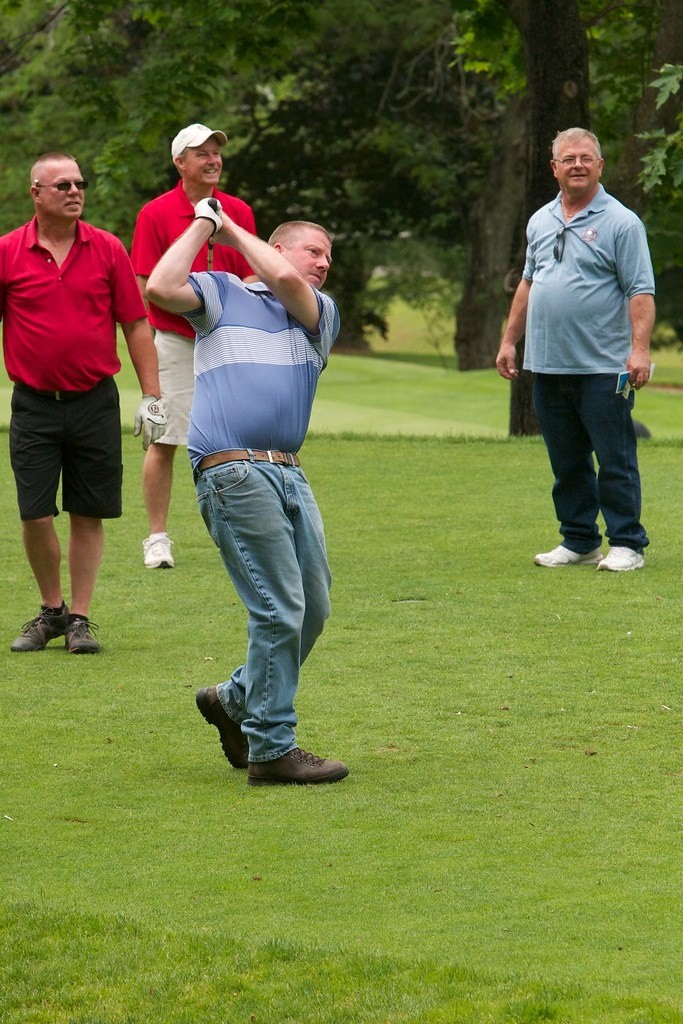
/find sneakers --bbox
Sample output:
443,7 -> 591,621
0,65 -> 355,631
246,747 -> 349,786
534,545 -> 602,568
141,533 -> 174,569
196,686 -> 250,769
10,606 -> 69,652
597,546 -> 645,571
63,615 -> 101,653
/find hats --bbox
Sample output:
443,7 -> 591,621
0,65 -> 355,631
171,123 -> 228,162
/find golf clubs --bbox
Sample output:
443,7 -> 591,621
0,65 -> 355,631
207,198 -> 218,271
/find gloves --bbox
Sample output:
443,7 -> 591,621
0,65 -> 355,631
193,197 -> 223,233
132,395 -> 168,452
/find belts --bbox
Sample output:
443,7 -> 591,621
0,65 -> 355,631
199,449 -> 300,471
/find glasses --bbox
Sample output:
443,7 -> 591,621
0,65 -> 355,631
553,157 -> 601,167
553,226 -> 565,263
35,181 -> 89,190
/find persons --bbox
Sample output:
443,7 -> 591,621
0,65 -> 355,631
144,196 -> 348,785
130,124 -> 256,569
496,129 -> 655,570
0,152 -> 166,655
